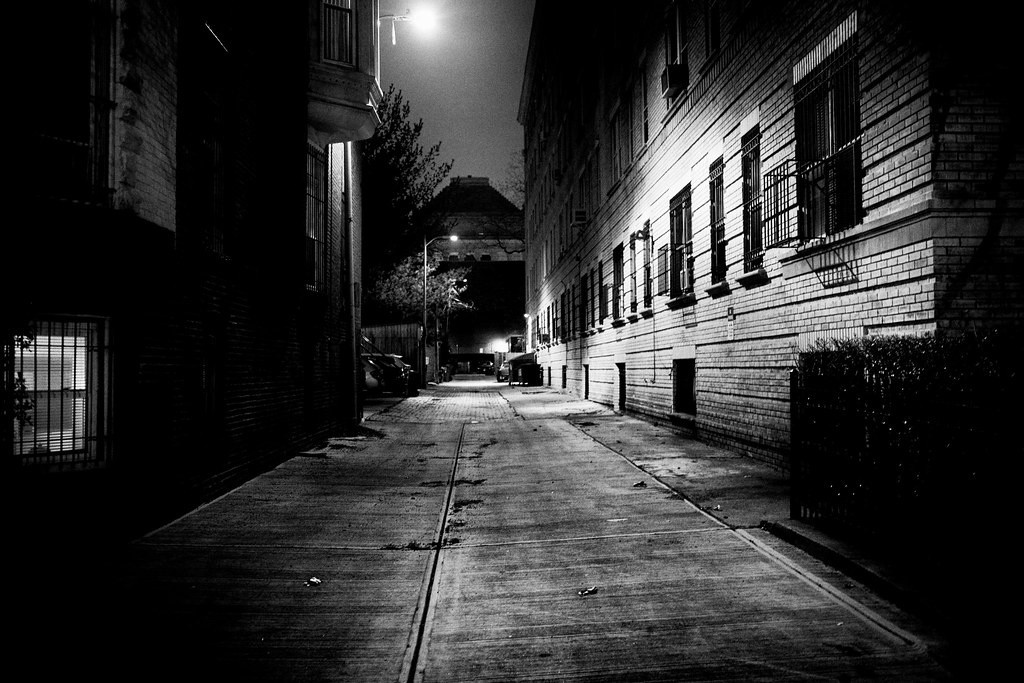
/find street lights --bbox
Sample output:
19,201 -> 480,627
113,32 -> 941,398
419,234 -> 460,387
